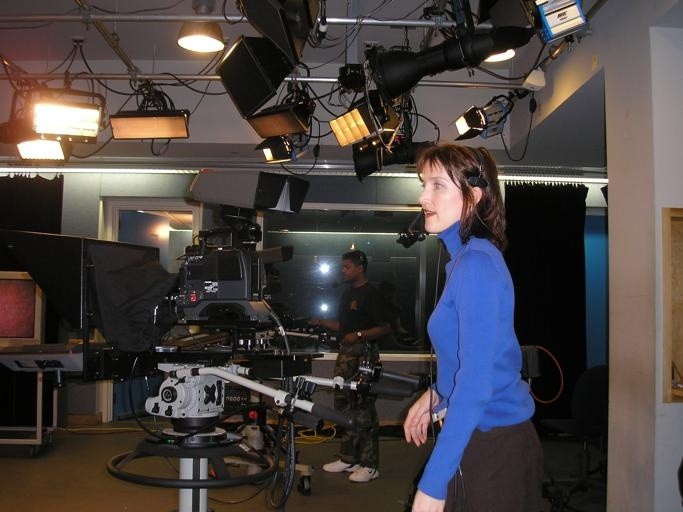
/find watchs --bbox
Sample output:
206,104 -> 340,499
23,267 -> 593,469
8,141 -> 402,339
356,331 -> 363,341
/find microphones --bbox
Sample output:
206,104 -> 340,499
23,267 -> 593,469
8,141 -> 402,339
402,228 -> 425,248
332,281 -> 347,288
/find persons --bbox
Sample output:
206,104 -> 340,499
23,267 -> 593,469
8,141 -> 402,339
403,144 -> 543,512
307,249 -> 395,483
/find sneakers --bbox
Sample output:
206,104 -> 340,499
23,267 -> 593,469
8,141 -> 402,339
348,467 -> 378,482
322,459 -> 360,473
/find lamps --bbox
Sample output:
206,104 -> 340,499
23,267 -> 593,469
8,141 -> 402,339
17,139 -> 68,160
177,1 -> 225,53
255,135 -> 295,164
448,101 -> 508,142
382,105 -> 402,132
328,90 -> 386,148
246,99 -> 316,137
28,88 -> 105,145
110,109 -> 190,141
484,48 -> 515,62
